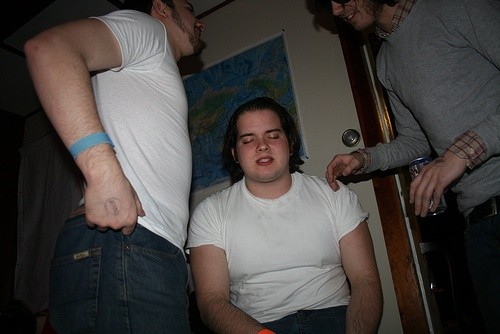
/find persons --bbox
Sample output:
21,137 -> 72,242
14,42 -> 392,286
312,0 -> 500,334
187,96 -> 383,334
22,0 -> 206,333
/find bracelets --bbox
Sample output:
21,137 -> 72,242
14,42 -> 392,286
257,329 -> 276,334
68,133 -> 115,161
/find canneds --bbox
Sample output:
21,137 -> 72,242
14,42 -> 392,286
408,157 -> 447,215
